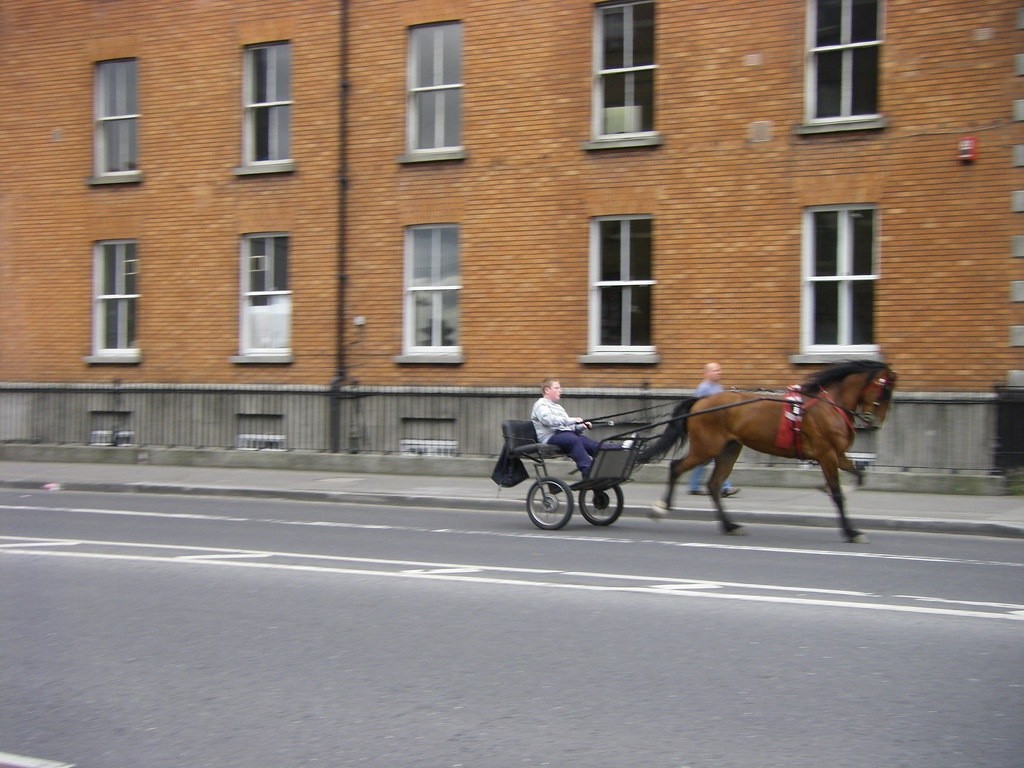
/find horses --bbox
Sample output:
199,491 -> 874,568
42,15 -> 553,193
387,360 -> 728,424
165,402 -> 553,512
628,356 -> 899,544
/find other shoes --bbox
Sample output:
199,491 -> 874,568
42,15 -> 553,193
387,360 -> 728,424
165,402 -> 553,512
689,488 -> 710,496
621,433 -> 638,452
720,486 -> 738,498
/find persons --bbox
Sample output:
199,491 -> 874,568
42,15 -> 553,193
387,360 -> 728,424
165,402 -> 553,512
530,378 -> 638,480
687,363 -> 740,498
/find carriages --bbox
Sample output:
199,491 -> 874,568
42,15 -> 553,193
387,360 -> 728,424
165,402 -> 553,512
496,356 -> 898,546
